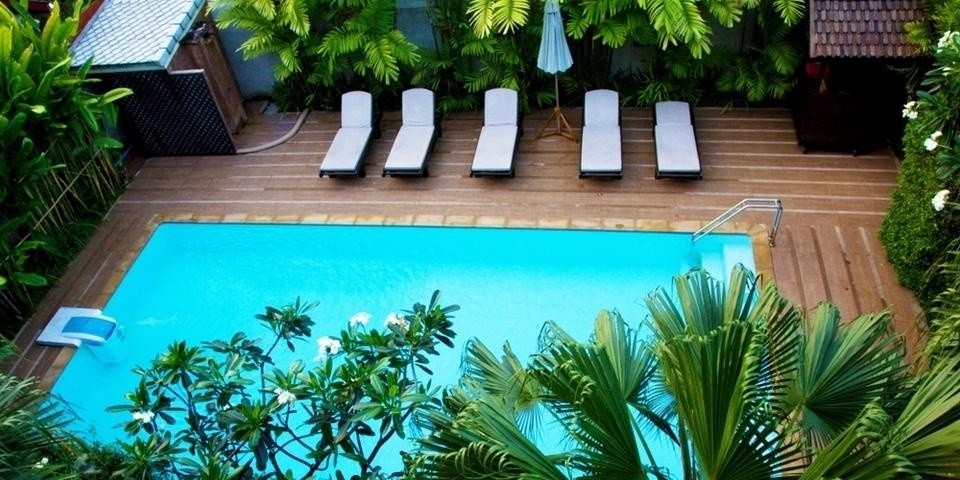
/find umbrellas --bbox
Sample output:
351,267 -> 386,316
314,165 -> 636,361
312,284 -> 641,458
536,0 -> 574,135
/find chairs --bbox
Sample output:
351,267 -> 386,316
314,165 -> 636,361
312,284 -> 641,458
470,87 -> 525,178
319,91 -> 378,179
652,100 -> 704,180
578,89 -> 624,180
381,87 -> 442,179
